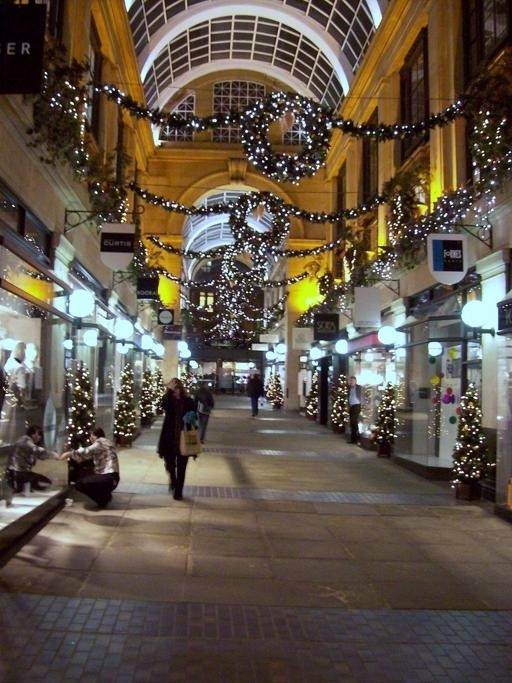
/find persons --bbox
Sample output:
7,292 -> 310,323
156,377 -> 201,502
5,424 -> 62,493
245,372 -> 267,417
345,375 -> 366,446
58,427 -> 120,510
192,376 -> 214,444
0,338 -> 30,442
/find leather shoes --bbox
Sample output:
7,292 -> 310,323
170,479 -> 183,500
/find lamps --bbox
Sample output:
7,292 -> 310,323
266,300 -> 494,364
53,285 -> 199,369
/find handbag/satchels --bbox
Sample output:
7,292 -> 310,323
179,423 -> 202,456
